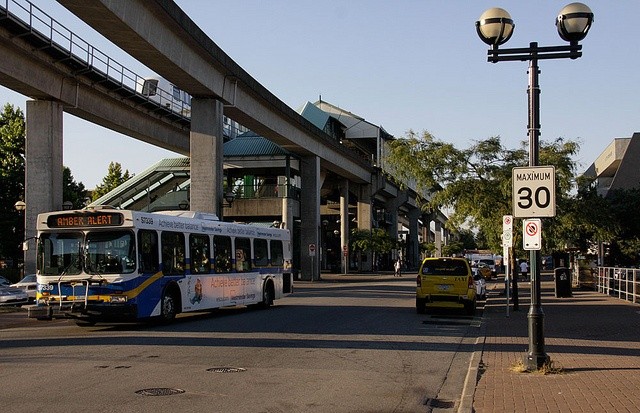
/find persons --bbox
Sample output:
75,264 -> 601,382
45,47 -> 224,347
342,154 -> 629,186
393,258 -> 401,277
103,252 -> 123,273
519,260 -> 529,282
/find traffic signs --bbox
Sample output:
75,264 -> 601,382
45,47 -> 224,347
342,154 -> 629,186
344,244 -> 347,256
503,215 -> 512,265
513,166 -> 556,218
523,220 -> 541,250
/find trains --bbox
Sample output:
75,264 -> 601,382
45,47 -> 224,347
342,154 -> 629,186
135,76 -> 251,141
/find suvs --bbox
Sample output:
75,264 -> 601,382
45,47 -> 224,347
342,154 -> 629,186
474,259 -> 497,278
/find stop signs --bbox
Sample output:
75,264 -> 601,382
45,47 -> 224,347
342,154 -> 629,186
309,244 -> 316,256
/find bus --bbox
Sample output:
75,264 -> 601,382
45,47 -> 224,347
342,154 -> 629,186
36,205 -> 293,328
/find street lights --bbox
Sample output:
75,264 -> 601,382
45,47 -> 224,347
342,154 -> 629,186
477,3 -> 594,373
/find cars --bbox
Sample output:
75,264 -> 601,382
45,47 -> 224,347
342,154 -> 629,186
0,277 -> 28,307
10,274 -> 37,307
471,268 -> 487,300
475,263 -> 491,281
416,257 -> 481,316
